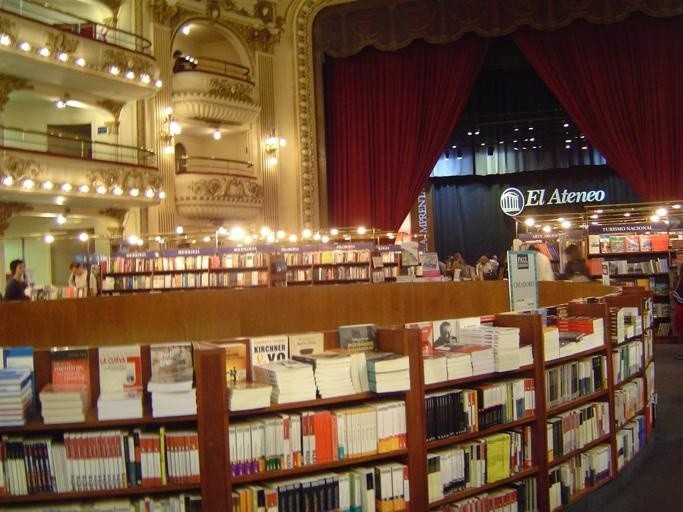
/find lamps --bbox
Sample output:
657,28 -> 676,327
2,173 -> 166,199
263,126 -> 286,164
162,104 -> 183,156
56,94 -> 70,110
1,31 -> 166,90
213,127 -> 223,142
51,195 -> 72,226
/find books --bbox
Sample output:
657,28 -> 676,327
652,234 -> 682,337
1,235 -> 656,512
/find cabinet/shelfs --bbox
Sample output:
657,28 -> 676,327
0,227 -> 683,512
